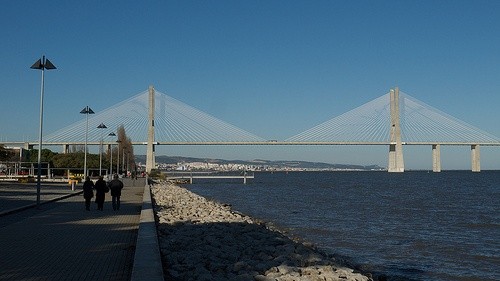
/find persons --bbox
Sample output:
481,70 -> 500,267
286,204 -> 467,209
135,172 -> 138,180
108,174 -> 123,210
83,176 -> 94,211
94,176 -> 108,211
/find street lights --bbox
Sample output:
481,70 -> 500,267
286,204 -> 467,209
116,139 -> 122,178
126,151 -> 130,173
79,106 -> 95,178
107,132 -> 116,180
96,122 -> 107,176
121,146 -> 126,175
31,55 -> 57,202
